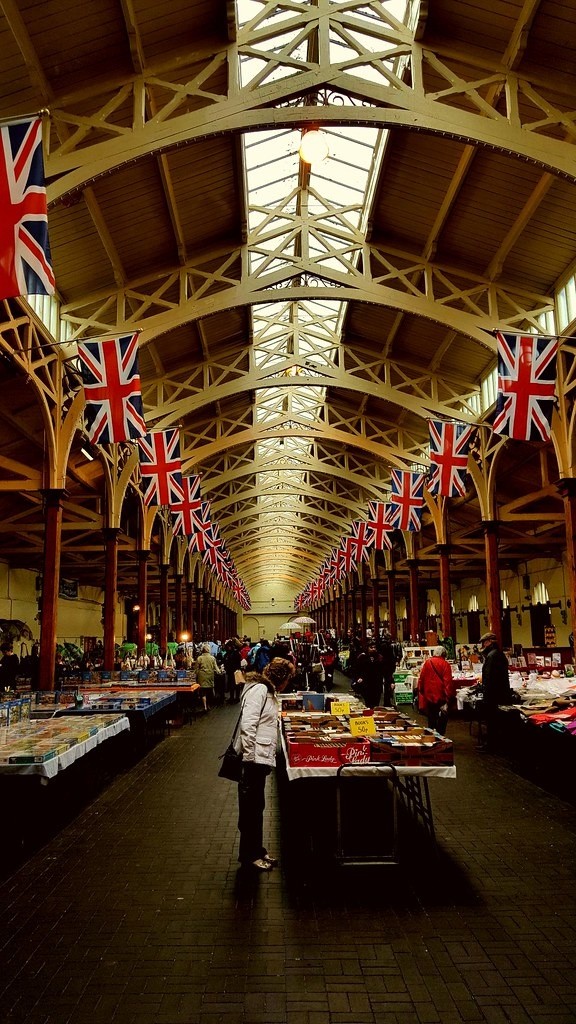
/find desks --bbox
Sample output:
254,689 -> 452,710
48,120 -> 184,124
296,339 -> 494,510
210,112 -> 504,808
29,690 -> 178,752
272,693 -> 456,900
79,680 -> 201,713
0,718 -> 130,775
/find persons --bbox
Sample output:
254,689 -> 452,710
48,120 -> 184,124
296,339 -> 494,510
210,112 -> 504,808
233,656 -> 296,872
0,628 -> 396,712
417,645 -> 455,736
475,632 -> 511,754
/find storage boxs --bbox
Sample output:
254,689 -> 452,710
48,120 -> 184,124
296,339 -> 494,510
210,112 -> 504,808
0,669 -> 198,764
281,693 -> 455,768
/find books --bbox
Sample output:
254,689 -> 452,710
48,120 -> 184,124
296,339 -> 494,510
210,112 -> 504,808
0,690 -> 176,764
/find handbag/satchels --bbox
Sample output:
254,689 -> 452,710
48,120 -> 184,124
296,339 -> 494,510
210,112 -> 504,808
234,669 -> 246,685
218,744 -> 244,782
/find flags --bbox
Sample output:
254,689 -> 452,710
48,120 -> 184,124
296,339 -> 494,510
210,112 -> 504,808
77,332 -> 251,612
427,420 -> 473,498
0,116 -> 56,300
492,332 -> 559,443
294,468 -> 424,611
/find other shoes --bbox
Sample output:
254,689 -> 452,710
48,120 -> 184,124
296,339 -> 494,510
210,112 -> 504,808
225,698 -> 240,703
253,859 -> 273,869
263,854 -> 277,863
475,745 -> 494,753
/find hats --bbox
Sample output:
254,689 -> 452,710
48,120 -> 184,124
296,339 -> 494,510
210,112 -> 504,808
479,633 -> 497,642
